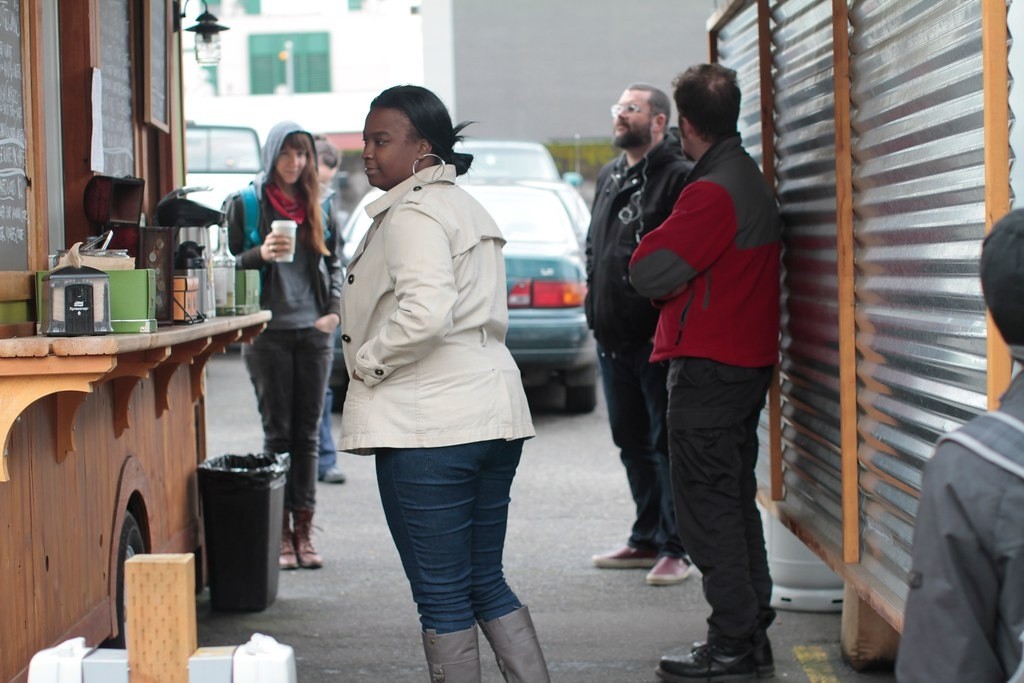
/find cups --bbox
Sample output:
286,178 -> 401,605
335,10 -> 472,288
271,219 -> 297,263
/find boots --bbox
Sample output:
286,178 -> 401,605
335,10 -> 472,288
421,619 -> 482,683
279,510 -> 324,570
479,605 -> 550,683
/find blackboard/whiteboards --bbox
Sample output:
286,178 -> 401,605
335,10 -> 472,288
89,0 -> 142,185
144,0 -> 173,135
0,0 -> 51,301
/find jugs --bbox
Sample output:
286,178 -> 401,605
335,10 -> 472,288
173,242 -> 206,322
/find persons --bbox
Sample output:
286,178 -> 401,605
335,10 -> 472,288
219,120 -> 349,569
336,86 -> 550,683
895,209 -> 1024,683
584,83 -> 696,585
630,63 -> 780,683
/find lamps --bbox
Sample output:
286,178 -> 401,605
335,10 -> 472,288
172,0 -> 231,66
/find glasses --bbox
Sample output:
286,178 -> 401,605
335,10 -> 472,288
611,105 -> 642,118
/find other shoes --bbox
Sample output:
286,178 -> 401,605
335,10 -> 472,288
592,544 -> 658,567
646,554 -> 694,585
322,468 -> 345,483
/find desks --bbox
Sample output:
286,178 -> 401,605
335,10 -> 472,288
0,309 -> 272,483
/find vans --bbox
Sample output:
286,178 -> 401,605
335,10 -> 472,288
186,106 -> 267,249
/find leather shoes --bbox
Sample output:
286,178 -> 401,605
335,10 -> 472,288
655,640 -> 775,683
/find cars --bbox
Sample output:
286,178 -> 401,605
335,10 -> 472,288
333,141 -> 599,414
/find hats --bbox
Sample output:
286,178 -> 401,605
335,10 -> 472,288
981,208 -> 1024,362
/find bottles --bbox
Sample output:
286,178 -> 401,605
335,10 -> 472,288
211,219 -> 236,316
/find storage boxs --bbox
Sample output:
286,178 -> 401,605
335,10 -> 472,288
34,269 -> 158,335
172,275 -> 197,323
203,268 -> 262,316
81,175 -> 180,327
175,268 -> 204,314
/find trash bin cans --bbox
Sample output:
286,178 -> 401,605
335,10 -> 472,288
197,453 -> 291,613
